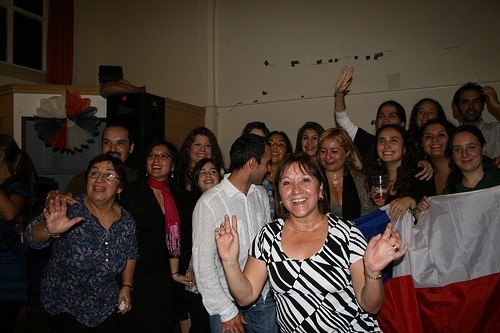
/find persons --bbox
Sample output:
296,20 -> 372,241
213,152 -> 409,333
227,66 -> 500,221
190,133 -> 278,333
0,119 -> 230,333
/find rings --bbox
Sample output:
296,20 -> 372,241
50,212 -> 55,215
393,245 -> 398,252
428,168 -> 432,172
398,209 -> 402,212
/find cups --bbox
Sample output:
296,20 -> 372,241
371,175 -> 389,200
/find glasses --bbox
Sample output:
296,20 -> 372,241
87,171 -> 121,181
148,152 -> 174,161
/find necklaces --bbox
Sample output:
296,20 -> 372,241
388,177 -> 395,182
91,205 -> 117,228
288,215 -> 324,233
327,172 -> 342,189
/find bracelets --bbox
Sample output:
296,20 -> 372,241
44,228 -> 61,239
365,271 -> 383,279
171,271 -> 179,276
123,285 -> 134,291
